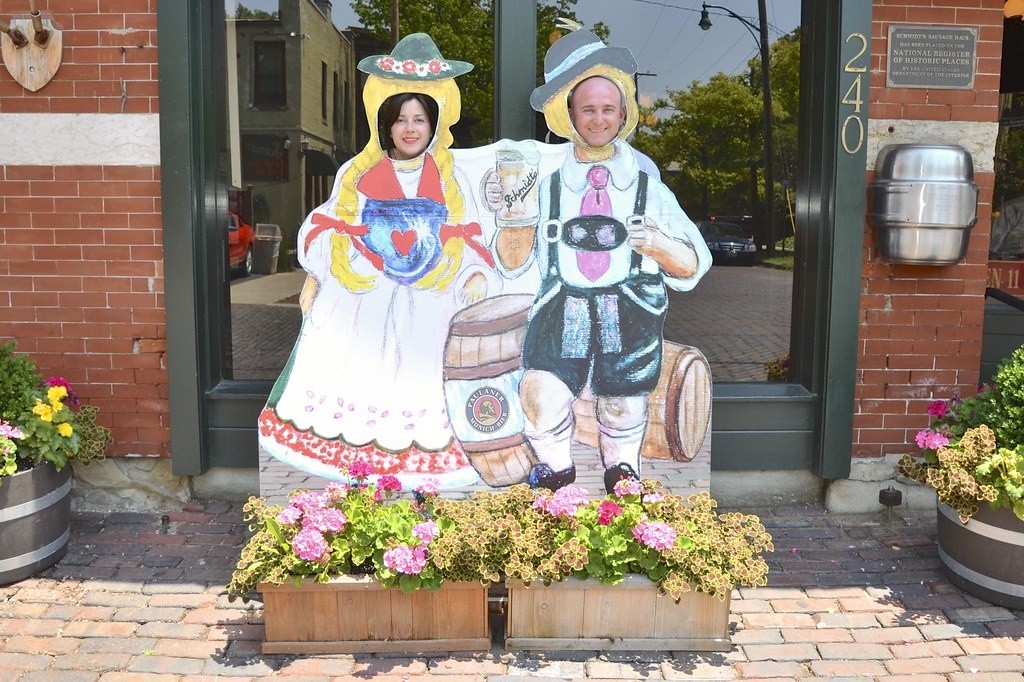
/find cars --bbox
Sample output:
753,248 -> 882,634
694,220 -> 757,265
228,209 -> 258,277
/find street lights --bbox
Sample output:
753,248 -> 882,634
697,1 -> 777,251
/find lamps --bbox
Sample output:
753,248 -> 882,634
297,135 -> 310,159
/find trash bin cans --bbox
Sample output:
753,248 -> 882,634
251,223 -> 280,274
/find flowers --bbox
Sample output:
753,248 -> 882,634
0,339 -> 114,487
899,344 -> 1024,524
218,461 -> 774,603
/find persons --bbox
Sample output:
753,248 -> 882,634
381,93 -> 439,160
568,76 -> 626,148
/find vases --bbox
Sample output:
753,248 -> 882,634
505,574 -> 733,651
937,495 -> 1024,609
0,461 -> 71,585
257,574 -> 493,654
